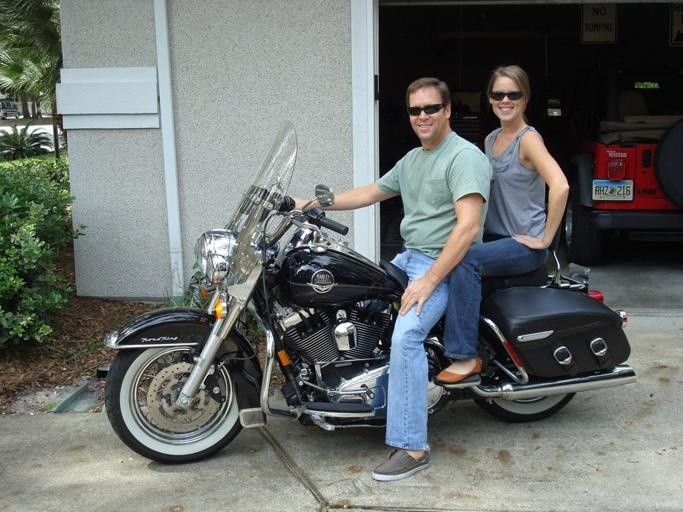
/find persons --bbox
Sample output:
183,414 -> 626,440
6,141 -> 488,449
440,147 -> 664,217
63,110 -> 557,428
285,77 -> 494,482
435,65 -> 570,384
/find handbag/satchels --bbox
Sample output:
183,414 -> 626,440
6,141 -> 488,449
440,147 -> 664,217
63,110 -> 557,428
492,284 -> 632,380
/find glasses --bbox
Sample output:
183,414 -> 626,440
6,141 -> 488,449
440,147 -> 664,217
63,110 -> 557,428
489,90 -> 523,102
407,102 -> 443,117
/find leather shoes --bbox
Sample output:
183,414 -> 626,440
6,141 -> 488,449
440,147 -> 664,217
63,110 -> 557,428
435,356 -> 482,383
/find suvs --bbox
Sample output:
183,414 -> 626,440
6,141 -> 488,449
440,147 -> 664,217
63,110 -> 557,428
565,73 -> 683,264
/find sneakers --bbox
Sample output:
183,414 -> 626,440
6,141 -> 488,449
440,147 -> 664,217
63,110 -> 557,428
371,447 -> 431,481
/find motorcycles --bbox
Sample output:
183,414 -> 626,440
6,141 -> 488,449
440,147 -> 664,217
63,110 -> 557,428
103,122 -> 637,465
